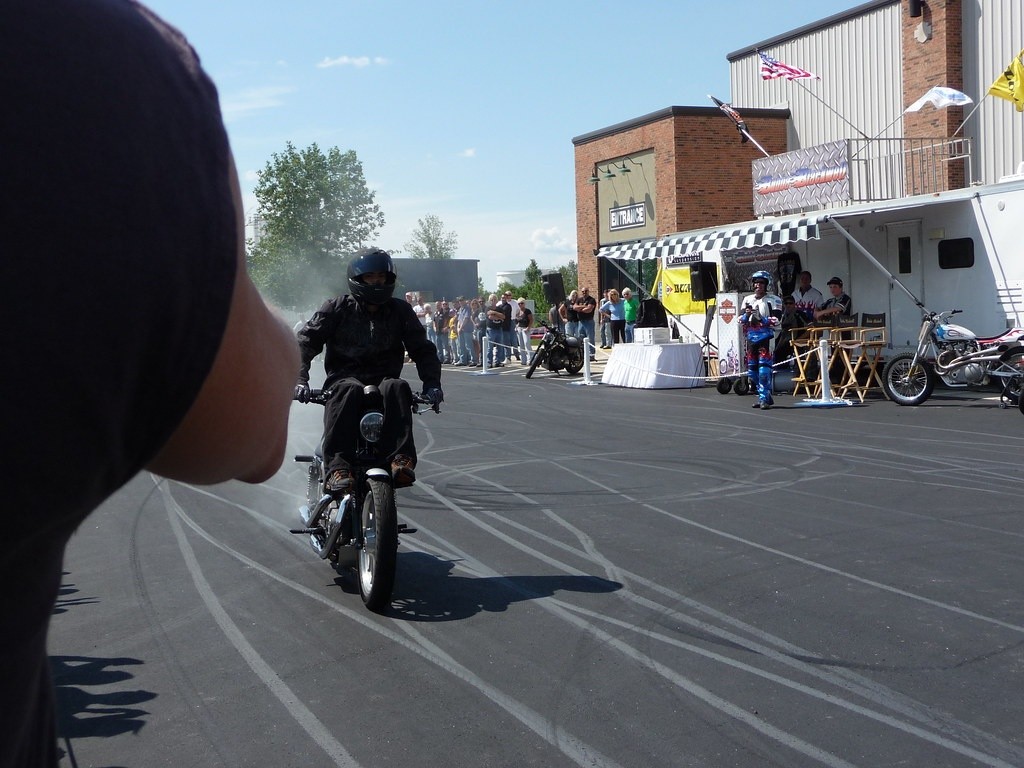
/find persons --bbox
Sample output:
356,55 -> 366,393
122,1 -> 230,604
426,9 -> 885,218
738,270 -> 782,410
295,247 -> 442,497
772,271 -> 852,397
398,286 -> 641,369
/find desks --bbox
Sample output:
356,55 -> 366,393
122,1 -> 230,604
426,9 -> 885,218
702,354 -> 718,358
601,343 -> 705,388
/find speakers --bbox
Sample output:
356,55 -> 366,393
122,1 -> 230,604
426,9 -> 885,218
690,261 -> 719,302
542,273 -> 566,305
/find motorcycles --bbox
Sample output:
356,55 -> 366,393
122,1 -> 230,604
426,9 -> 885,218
290,386 -> 432,610
881,309 -> 1024,406
526,317 -> 584,379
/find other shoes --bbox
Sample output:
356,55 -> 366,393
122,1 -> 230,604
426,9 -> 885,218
439,356 -> 531,368
752,400 -> 762,407
760,398 -> 774,408
600,345 -> 606,348
603,346 -> 611,349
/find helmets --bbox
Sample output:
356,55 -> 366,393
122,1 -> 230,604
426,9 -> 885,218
751,271 -> 774,291
347,248 -> 397,305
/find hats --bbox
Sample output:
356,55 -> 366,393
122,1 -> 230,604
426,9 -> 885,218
827,277 -> 843,286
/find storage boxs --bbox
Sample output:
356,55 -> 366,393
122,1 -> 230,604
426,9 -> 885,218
710,359 -> 719,376
634,327 -> 670,344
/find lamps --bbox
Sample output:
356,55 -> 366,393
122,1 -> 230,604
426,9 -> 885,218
589,156 -> 642,185
908,0 -> 925,17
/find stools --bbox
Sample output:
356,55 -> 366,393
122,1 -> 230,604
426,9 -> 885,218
788,312 -> 891,402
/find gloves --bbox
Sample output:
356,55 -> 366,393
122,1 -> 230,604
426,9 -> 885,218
422,388 -> 444,414
293,384 -> 311,405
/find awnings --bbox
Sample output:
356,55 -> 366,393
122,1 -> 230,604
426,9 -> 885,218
594,198 -> 976,355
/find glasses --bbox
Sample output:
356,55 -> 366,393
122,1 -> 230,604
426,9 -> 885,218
783,302 -> 794,305
518,303 -> 523,305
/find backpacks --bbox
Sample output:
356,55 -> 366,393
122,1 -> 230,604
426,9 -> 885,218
636,299 -> 667,327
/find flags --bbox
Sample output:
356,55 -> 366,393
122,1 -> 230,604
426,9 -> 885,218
986,49 -> 1024,112
759,52 -> 821,82
710,95 -> 750,144
903,86 -> 973,114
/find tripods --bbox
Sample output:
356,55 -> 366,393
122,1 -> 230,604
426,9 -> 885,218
701,300 -> 719,380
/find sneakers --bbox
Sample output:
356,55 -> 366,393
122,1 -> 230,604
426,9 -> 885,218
325,470 -> 354,492
392,454 -> 415,485
